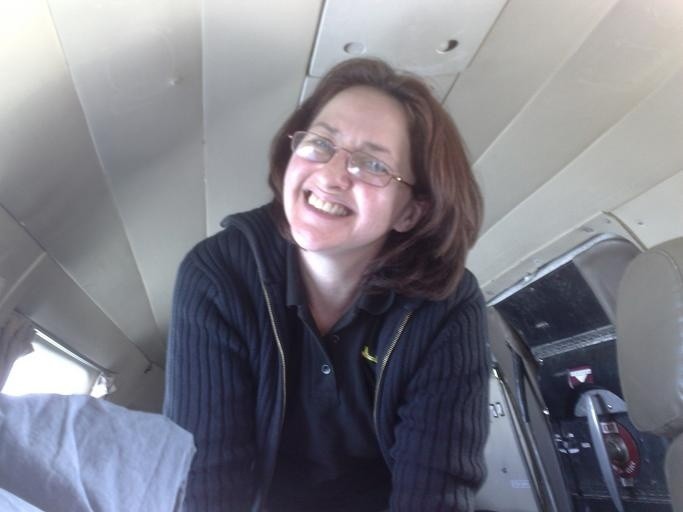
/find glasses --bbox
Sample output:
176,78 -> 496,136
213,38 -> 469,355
287,130 -> 415,189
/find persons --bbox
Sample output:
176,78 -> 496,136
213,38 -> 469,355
166,56 -> 489,511
